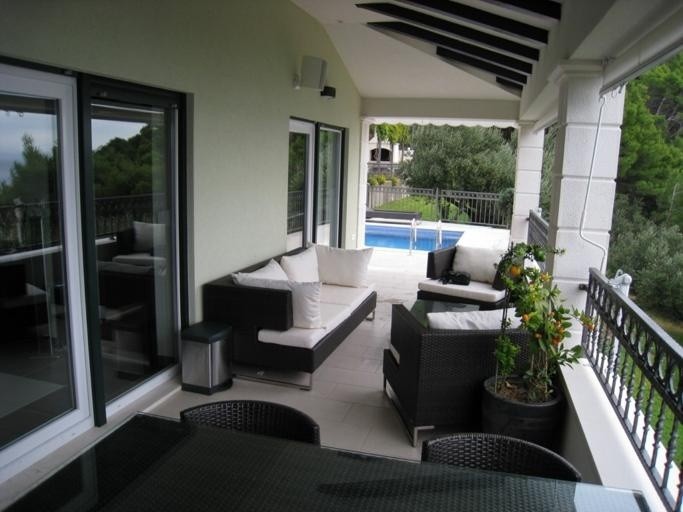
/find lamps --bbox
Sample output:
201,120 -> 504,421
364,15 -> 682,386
289,54 -> 328,91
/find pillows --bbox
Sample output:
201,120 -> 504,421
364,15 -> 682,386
230,242 -> 373,329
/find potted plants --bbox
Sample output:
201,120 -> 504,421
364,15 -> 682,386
478,241 -> 596,452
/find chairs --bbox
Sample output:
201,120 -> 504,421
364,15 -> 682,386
421,431 -> 581,481
178,399 -> 319,444
0,220 -> 169,361
382,244 -> 538,447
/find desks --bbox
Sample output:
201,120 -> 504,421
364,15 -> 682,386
3,409 -> 648,510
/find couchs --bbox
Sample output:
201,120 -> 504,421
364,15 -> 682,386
201,244 -> 378,392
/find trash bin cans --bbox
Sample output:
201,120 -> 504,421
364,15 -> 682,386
181,318 -> 233,395
